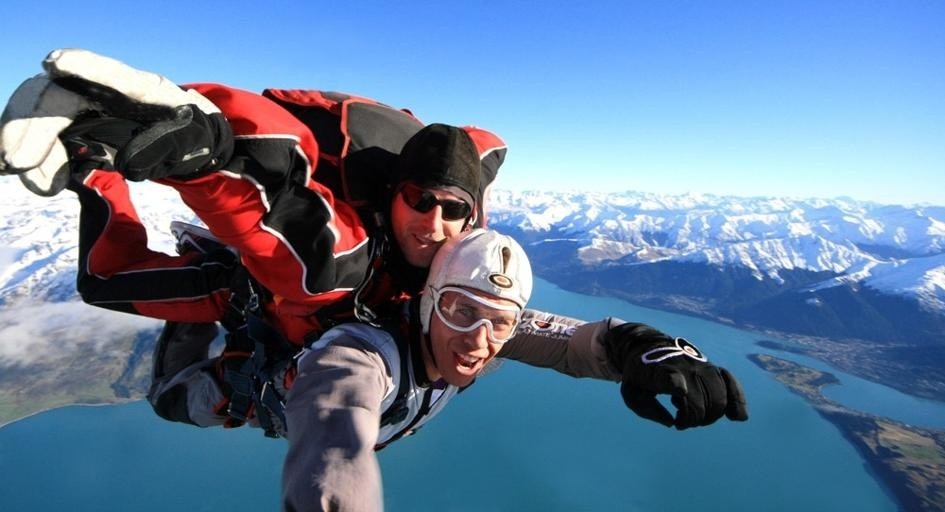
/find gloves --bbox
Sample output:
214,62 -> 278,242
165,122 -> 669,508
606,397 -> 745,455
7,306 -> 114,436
1,47 -> 234,195
603,323 -> 747,431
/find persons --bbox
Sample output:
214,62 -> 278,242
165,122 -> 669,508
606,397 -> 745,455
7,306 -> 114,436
1,47 -> 509,346
146,220 -> 751,512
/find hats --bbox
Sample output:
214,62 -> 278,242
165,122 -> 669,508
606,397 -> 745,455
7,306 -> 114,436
399,123 -> 480,199
420,228 -> 533,335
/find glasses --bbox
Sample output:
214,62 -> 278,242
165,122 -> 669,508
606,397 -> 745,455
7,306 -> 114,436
430,286 -> 522,343
402,184 -> 471,221
